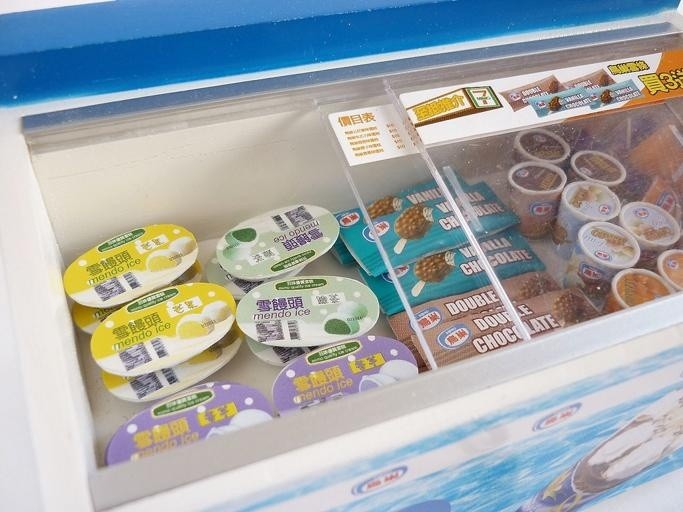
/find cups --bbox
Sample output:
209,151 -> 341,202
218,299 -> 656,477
505,131 -> 680,316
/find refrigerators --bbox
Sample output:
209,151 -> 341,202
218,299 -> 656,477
1,1 -> 683,512
